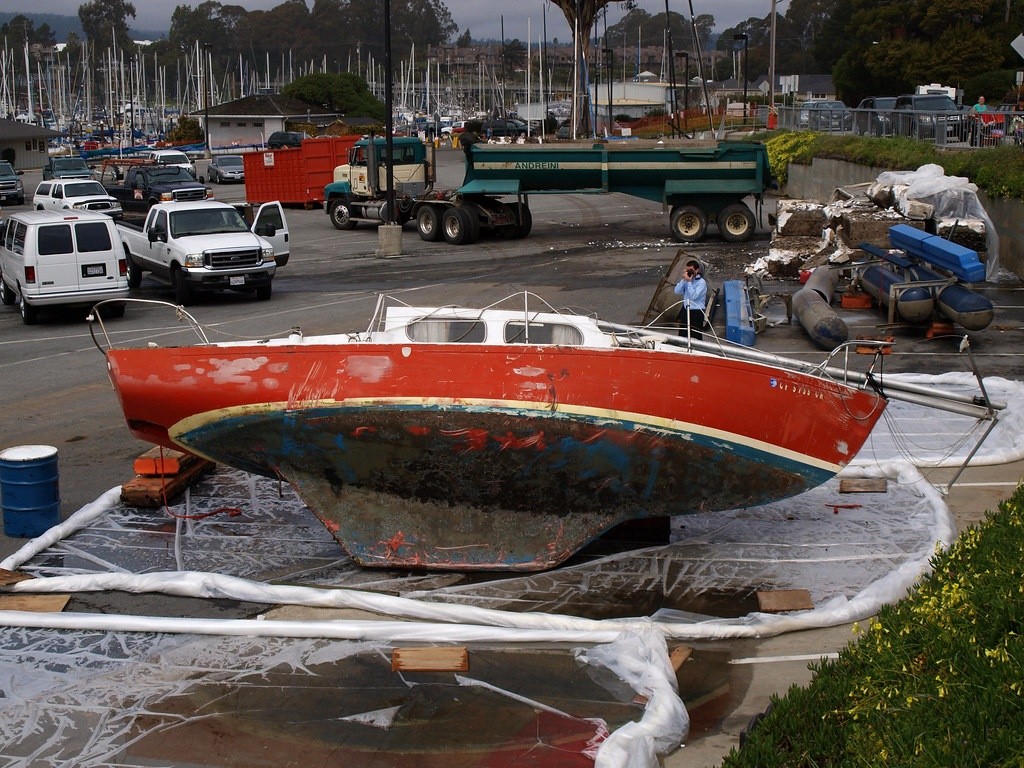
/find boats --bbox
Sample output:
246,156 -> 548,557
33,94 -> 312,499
104,339 -> 886,577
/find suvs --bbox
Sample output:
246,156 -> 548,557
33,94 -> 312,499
0,159 -> 26,206
853,95 -> 896,136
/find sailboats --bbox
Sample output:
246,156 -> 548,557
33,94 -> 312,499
202,286 -> 1008,497
0,26 -> 207,156
391,43 -> 521,117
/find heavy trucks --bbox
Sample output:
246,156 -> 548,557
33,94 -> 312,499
322,131 -> 777,258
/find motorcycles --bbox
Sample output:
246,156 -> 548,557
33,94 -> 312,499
1016,117 -> 1024,152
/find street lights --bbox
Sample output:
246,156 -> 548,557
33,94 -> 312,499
602,47 -> 616,135
675,51 -> 690,132
734,32 -> 751,126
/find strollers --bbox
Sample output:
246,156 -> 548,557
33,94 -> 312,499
972,113 -> 1004,148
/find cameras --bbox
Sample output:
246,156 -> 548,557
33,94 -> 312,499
687,269 -> 694,277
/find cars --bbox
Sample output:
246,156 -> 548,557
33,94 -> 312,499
892,94 -> 968,142
795,100 -> 853,132
267,131 -> 313,149
207,155 -> 245,184
395,120 -> 632,141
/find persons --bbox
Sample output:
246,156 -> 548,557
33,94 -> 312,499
1010,96 -> 1024,147
968,96 -> 988,148
674,260 -> 707,341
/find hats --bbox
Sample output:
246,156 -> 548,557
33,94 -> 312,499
1018,97 -> 1024,102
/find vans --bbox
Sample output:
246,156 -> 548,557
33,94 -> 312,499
0,199 -> 131,323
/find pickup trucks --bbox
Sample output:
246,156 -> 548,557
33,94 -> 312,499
103,164 -> 216,214
996,104 -> 1024,114
148,150 -> 197,180
32,179 -> 125,222
42,156 -> 96,181
116,197 -> 288,306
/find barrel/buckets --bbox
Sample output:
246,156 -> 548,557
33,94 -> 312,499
0,445 -> 61,536
